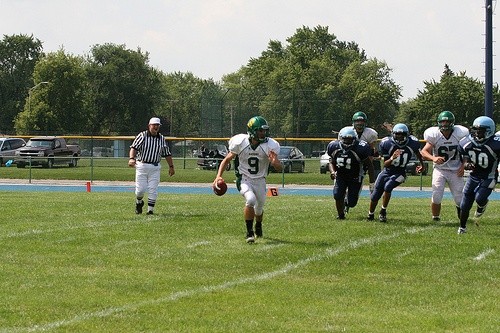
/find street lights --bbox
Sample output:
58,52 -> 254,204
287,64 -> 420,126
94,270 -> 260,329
28,82 -> 49,117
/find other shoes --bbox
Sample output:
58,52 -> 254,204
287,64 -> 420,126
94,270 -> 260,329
245,231 -> 255,243
337,214 -> 346,219
344,198 -> 350,212
255,223 -> 263,236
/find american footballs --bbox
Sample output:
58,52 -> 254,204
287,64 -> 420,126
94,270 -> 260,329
214,179 -> 227,196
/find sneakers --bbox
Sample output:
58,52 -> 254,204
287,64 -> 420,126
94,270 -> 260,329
367,213 -> 374,220
135,200 -> 144,214
474,203 -> 487,217
456,226 -> 467,235
379,210 -> 387,223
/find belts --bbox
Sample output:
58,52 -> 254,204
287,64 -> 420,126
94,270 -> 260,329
138,160 -> 159,164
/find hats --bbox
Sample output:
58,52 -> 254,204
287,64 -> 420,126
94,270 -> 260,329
149,118 -> 160,125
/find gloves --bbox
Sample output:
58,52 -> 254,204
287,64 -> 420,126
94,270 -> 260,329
331,172 -> 335,180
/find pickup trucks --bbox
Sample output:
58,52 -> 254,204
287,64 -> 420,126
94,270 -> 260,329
14,137 -> 81,169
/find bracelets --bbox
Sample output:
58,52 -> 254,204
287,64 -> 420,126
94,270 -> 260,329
169,165 -> 174,168
374,157 -> 376,160
130,157 -> 134,159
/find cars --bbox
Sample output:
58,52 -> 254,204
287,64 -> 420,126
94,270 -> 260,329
0,137 -> 26,166
406,156 -> 429,176
268,146 -> 306,173
320,150 -> 332,173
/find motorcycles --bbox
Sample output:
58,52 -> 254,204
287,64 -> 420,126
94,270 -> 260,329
206,145 -> 232,171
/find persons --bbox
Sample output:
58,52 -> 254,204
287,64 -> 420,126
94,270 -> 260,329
128,117 -> 175,215
457,116 -> 500,235
365,123 -> 424,222
420,111 -> 469,223
213,116 -> 282,243
327,112 -> 381,220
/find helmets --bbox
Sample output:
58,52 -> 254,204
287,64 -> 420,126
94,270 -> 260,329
352,112 -> 367,131
338,126 -> 358,153
392,123 -> 408,147
247,116 -> 270,143
494,131 -> 500,141
469,116 -> 495,147
437,111 -> 456,131
